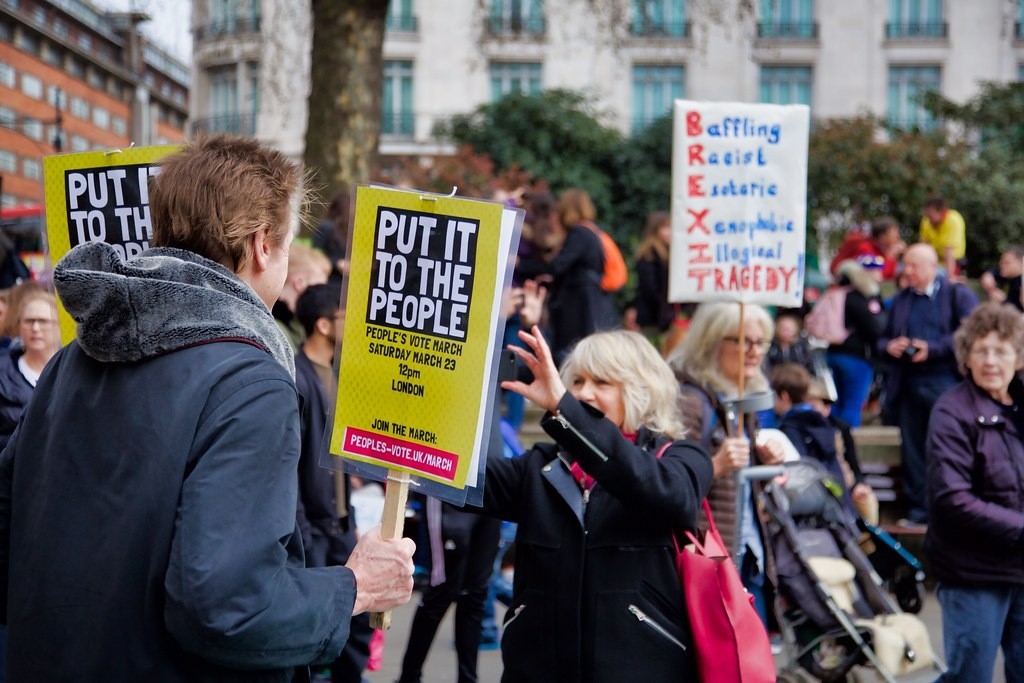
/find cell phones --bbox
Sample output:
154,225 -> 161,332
497,351 -> 518,381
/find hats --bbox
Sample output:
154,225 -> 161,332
857,254 -> 885,269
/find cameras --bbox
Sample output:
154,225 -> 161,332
901,341 -> 920,359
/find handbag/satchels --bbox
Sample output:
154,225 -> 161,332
654,442 -> 777,683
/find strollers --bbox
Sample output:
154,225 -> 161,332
729,430 -> 949,683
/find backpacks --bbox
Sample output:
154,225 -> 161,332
580,220 -> 628,292
804,285 -> 855,344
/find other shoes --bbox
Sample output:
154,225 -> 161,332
897,518 -> 928,529
768,632 -> 800,653
451,636 -> 502,650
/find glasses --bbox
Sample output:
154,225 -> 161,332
724,336 -> 771,355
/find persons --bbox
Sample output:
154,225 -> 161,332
1,134 -> 1024,683
